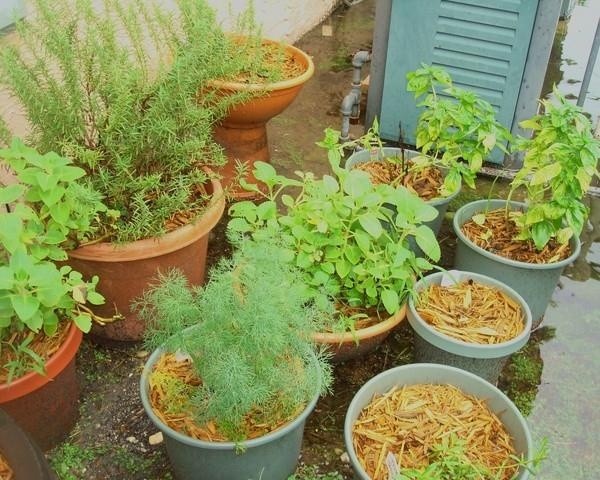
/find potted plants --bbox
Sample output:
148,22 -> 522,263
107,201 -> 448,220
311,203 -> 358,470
0,137 -> 105,451
453,79 -> 600,330
323,58 -> 500,255
0,1 -> 227,344
228,148 -> 441,362
127,233 -> 337,479
183,0 -> 316,200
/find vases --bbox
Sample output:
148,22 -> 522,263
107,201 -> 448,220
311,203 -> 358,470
341,362 -> 532,480
409,270 -> 534,386
